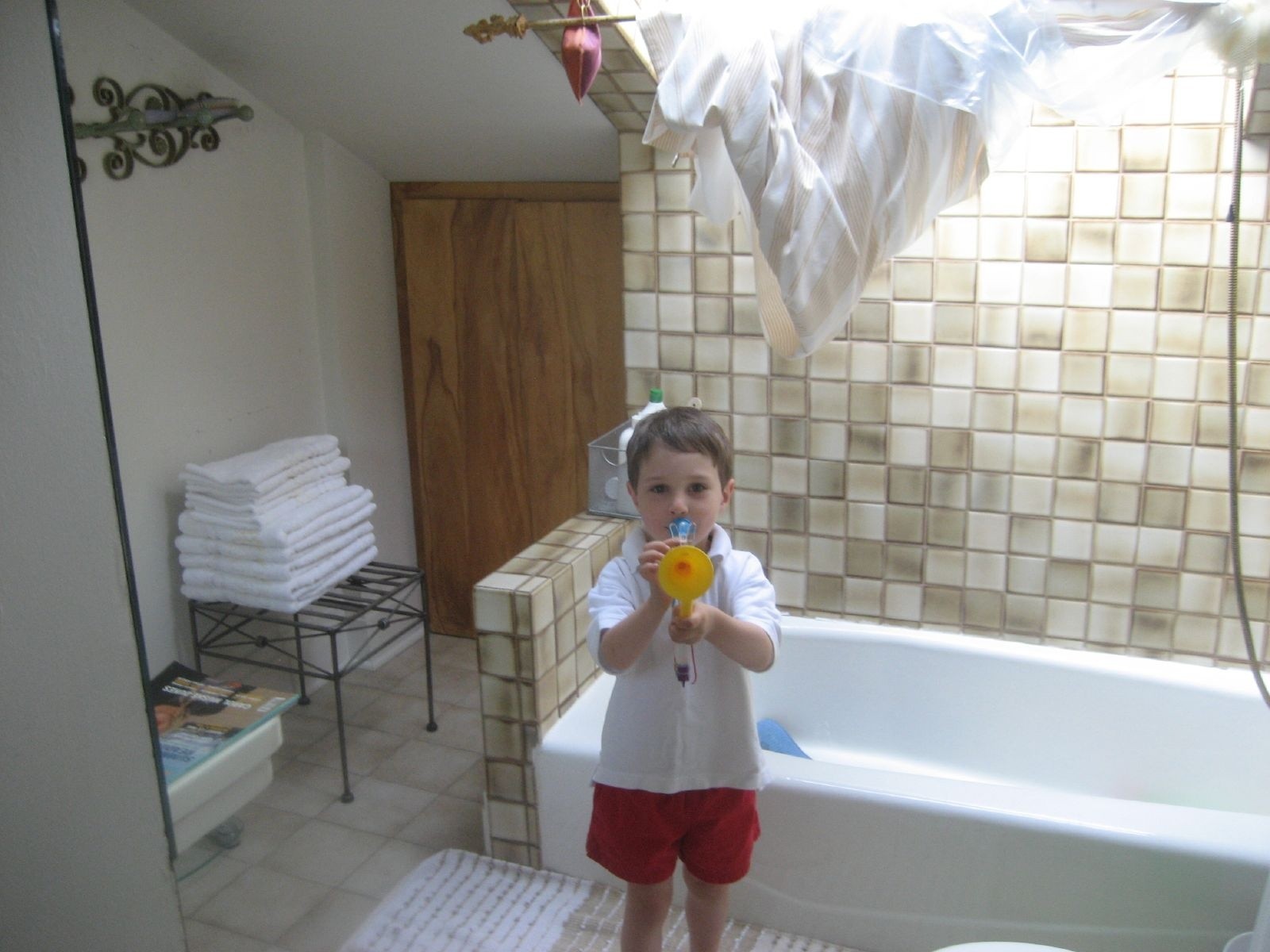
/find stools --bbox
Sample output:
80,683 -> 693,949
168,714 -> 283,853
190,563 -> 438,805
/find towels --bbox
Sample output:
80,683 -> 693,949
173,433 -> 380,615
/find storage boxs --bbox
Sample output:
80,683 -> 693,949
587,415 -> 645,521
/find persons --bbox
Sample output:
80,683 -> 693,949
582,405 -> 779,952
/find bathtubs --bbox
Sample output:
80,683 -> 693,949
527,613 -> 1269,952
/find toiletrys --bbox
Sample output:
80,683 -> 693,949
603,387 -> 703,517
